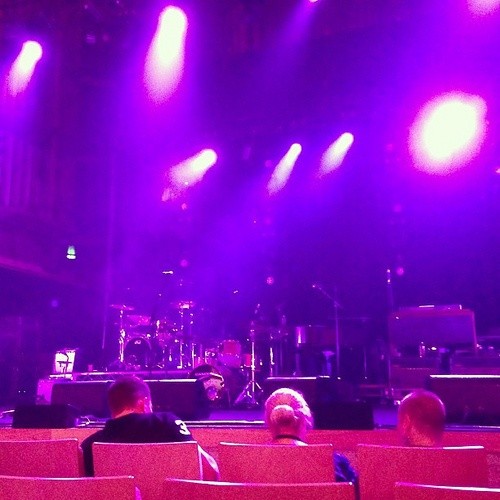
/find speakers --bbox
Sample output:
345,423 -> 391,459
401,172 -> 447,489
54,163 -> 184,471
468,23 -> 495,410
427,372 -> 500,426
141,377 -> 212,422
265,373 -> 331,412
53,378 -> 116,419
384,307 -> 476,367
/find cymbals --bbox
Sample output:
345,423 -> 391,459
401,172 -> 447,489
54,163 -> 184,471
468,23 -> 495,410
169,300 -> 195,310
109,304 -> 134,310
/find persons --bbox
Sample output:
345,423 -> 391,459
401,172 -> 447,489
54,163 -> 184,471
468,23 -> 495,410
264,388 -> 358,482
396,391 -> 447,447
81,374 -> 221,481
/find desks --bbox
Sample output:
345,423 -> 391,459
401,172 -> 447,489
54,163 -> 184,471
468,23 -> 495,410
37,370 -> 198,420
429,374 -> 500,426
262,376 -> 331,411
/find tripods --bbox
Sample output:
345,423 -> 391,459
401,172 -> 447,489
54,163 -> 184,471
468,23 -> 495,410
231,314 -> 264,407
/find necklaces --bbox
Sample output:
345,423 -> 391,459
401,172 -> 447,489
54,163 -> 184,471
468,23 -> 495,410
275,434 -> 302,443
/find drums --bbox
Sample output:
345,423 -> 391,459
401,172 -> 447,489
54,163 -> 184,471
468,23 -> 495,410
219,339 -> 242,366
193,357 -> 217,368
155,320 -> 178,341
125,336 -> 153,368
190,364 -> 235,405
239,354 -> 259,376
127,314 -> 152,337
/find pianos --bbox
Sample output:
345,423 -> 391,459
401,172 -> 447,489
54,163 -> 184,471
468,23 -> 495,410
292,323 -> 364,375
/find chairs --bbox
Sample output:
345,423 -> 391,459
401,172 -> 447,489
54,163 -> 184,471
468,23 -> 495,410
0,438 -> 500,500
